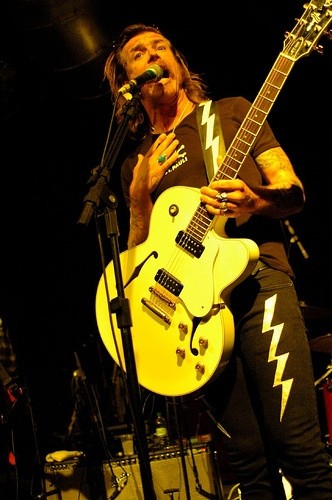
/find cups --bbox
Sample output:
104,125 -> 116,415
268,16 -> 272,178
121,434 -> 134,455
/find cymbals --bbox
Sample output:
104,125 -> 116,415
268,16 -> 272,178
305,331 -> 332,356
298,302 -> 330,319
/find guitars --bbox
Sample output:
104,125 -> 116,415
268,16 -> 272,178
94,0 -> 332,398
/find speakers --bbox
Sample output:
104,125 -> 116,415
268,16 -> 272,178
43,442 -> 222,500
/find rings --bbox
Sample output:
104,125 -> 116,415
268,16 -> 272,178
217,192 -> 227,201
158,155 -> 166,163
221,202 -> 227,209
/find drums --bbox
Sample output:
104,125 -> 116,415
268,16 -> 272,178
312,380 -> 332,449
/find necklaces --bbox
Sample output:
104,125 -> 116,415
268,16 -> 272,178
149,103 -> 189,134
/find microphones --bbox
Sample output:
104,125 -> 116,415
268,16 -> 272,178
110,481 -> 127,500
195,488 -> 219,500
118,63 -> 164,94
284,220 -> 309,259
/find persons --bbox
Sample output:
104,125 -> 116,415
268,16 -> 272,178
102,24 -> 332,500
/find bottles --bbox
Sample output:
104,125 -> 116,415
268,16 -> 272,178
145,420 -> 150,436
153,412 -> 169,446
